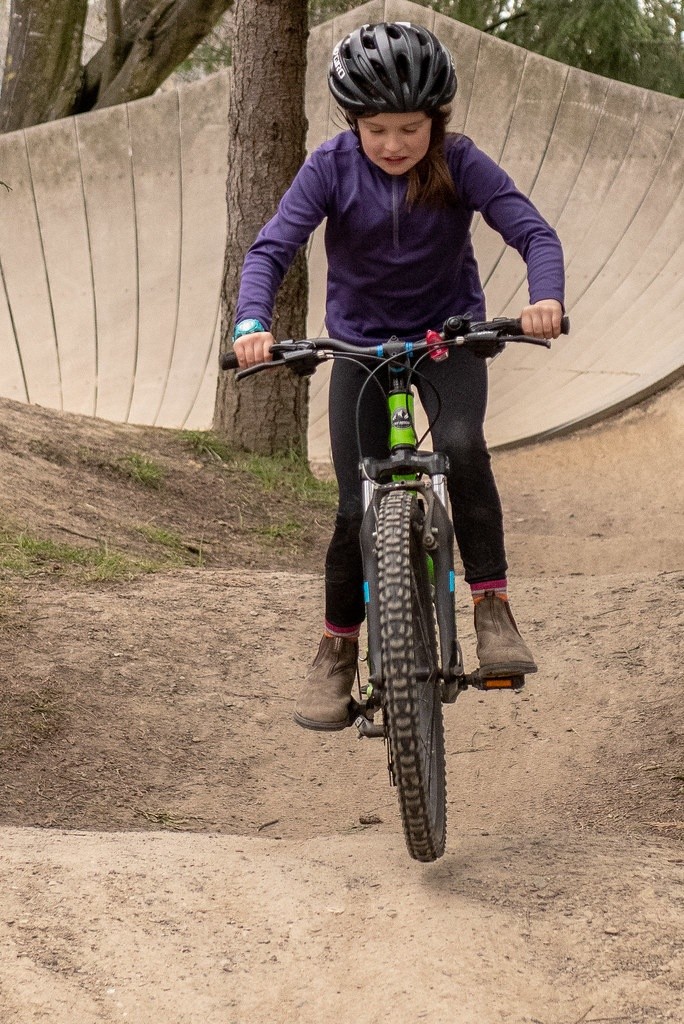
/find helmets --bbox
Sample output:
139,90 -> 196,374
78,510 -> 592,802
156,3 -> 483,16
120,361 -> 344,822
327,21 -> 457,112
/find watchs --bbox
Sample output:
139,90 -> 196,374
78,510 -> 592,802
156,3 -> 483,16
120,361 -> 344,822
232,319 -> 264,342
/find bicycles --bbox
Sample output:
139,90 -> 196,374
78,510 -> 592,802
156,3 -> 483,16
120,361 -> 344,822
219,311 -> 570,863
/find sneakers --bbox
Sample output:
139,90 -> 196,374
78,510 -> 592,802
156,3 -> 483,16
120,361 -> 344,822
473,594 -> 536,678
295,633 -> 360,731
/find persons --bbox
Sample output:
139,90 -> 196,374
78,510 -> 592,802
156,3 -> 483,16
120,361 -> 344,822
230,22 -> 568,734
